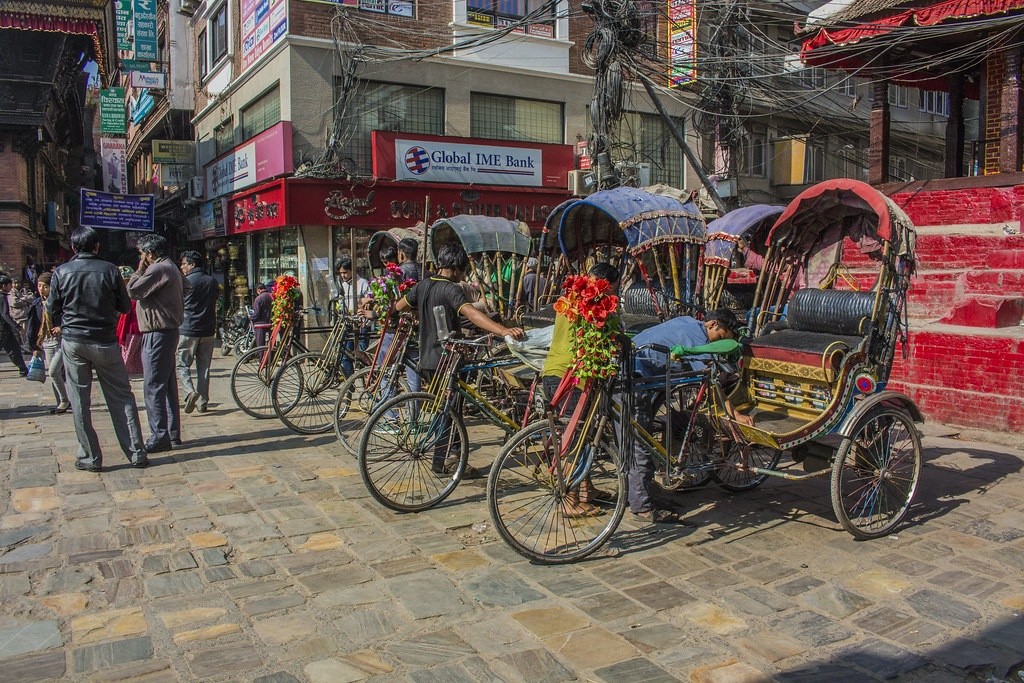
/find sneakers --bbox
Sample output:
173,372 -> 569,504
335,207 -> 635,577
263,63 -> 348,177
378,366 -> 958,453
375,423 -> 401,434
407,424 -> 418,435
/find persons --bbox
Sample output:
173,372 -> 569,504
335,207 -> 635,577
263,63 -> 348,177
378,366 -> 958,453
176,250 -> 220,414
394,245 -> 530,480
335,258 -> 373,394
25,271 -> 75,414
511,256 -> 551,320
123,233 -> 192,453
248,281 -> 276,368
540,260 -> 625,518
0,275 -> 29,377
46,224 -> 151,471
379,248 -> 398,279
369,237 -> 432,435
612,306 -> 739,526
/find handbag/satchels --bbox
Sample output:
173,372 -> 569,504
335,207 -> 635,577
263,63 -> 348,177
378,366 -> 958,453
25,353 -> 46,383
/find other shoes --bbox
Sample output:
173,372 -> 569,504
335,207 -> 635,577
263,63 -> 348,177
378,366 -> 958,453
196,401 -> 207,413
432,457 -> 476,477
170,439 -> 182,446
132,458 -> 150,468
75,459 -> 101,471
184,390 -> 199,414
21,344 -> 32,353
20,367 -> 29,377
142,442 -> 173,452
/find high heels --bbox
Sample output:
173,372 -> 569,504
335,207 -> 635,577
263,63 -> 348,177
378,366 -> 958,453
50,401 -> 71,413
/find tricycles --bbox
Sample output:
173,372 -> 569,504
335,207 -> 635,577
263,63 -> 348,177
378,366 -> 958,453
234,178 -> 928,562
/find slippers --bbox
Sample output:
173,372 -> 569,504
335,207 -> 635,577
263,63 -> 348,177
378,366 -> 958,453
630,503 -> 680,523
582,489 -> 612,503
561,501 -> 607,518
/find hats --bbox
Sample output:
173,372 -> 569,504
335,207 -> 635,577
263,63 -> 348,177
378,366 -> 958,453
527,258 -> 539,268
118,265 -> 135,279
37,272 -> 52,288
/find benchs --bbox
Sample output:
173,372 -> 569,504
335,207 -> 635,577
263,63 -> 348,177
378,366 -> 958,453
620,279 -> 695,334
748,288 -> 891,383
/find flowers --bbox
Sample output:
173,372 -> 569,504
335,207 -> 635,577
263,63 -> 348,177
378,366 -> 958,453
368,276 -> 398,333
554,274 -> 622,382
270,274 -> 300,330
